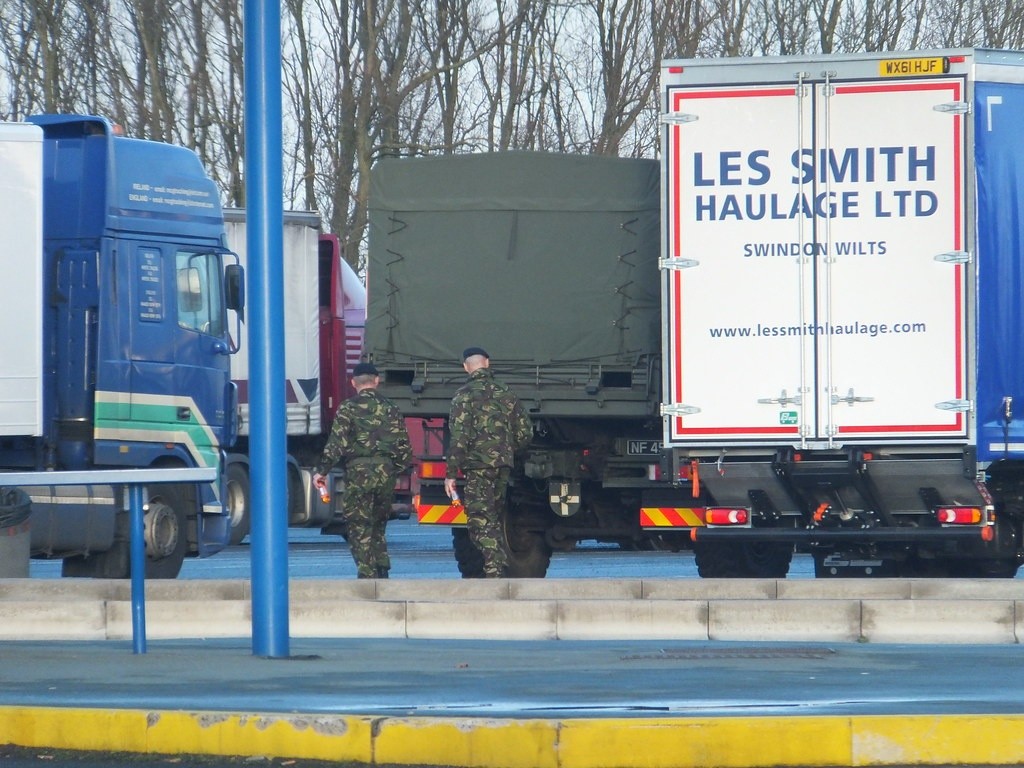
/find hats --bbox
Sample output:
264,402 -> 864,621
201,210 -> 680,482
463,347 -> 489,359
353,363 -> 378,376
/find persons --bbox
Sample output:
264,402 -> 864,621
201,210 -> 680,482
445,347 -> 534,578
313,363 -> 413,579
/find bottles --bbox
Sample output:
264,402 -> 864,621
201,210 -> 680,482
317,479 -> 331,503
448,483 -> 461,507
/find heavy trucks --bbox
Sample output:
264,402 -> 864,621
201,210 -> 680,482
0,114 -> 246,579
639,45 -> 1024,579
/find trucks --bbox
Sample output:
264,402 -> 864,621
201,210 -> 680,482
363,154 -> 662,578
175,207 -> 412,546
